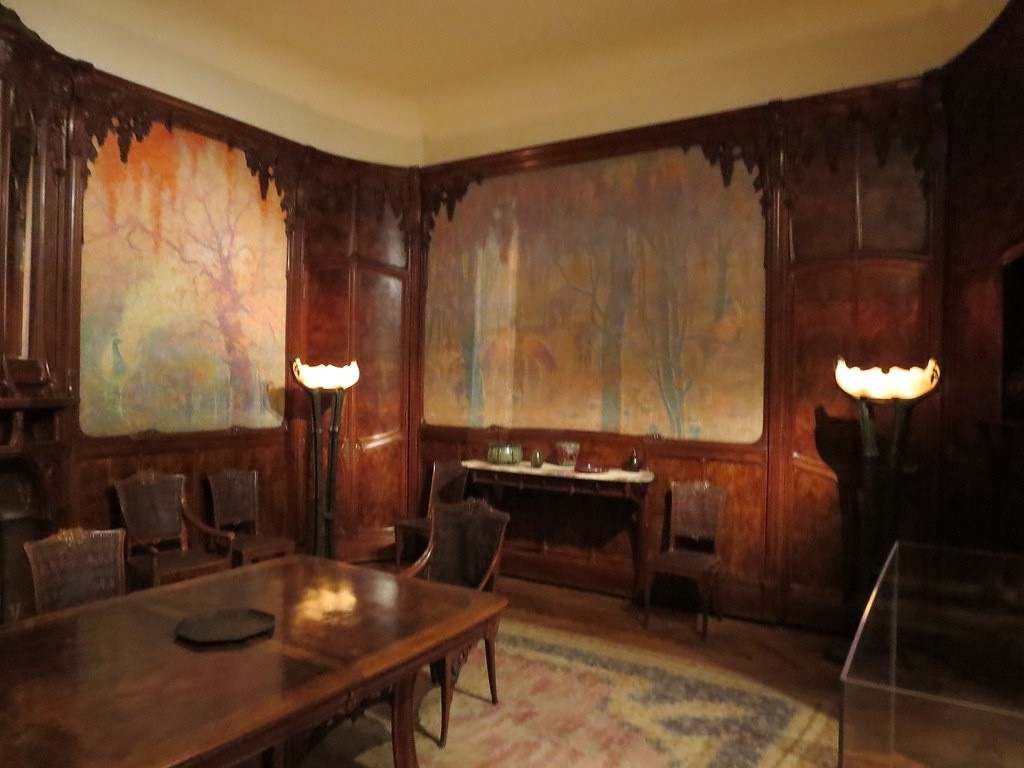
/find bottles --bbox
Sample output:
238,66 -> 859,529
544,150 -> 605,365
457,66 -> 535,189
626,446 -> 643,471
530,447 -> 543,468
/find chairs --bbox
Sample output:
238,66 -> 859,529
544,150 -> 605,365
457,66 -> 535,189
394,457 -> 468,568
114,468 -> 236,590
23,526 -> 127,617
206,468 -> 295,567
398,497 -> 510,747
641,480 -> 721,643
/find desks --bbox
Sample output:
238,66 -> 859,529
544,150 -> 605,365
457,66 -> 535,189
0,552 -> 509,768
460,458 -> 653,611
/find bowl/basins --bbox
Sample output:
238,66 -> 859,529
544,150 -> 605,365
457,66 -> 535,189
489,441 -> 523,464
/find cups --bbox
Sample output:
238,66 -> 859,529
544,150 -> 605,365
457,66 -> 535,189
556,440 -> 579,466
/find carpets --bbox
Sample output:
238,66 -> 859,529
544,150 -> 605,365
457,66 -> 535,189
297,617 -> 849,768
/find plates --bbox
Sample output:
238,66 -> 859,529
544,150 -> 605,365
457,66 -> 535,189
176,608 -> 276,645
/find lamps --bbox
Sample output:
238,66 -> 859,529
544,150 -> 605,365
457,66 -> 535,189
292,358 -> 361,559
824,357 -> 943,668
